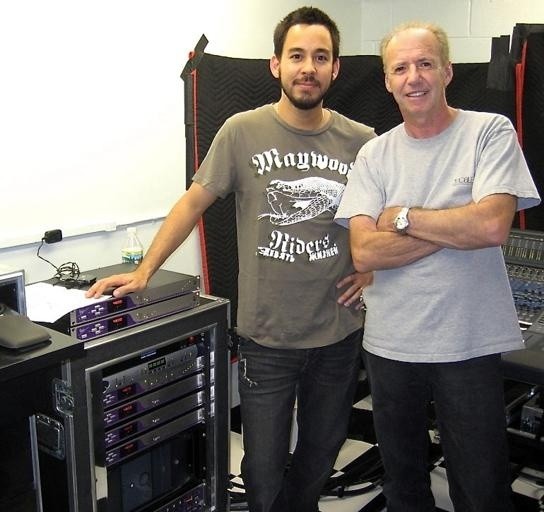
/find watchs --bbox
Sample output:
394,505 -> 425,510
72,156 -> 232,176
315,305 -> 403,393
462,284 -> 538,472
393,206 -> 411,236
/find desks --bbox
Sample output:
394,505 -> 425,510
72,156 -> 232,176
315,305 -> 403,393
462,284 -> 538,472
0,323 -> 84,383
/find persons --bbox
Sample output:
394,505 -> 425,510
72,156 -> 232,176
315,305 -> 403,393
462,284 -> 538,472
87,5 -> 379,512
333,22 -> 541,512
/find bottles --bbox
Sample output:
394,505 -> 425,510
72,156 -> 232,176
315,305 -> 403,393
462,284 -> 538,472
121,226 -> 144,266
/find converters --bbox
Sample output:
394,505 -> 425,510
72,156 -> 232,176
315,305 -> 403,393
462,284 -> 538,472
44,229 -> 62,243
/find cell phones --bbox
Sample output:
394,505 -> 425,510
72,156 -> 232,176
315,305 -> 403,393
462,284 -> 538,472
60,273 -> 97,284
